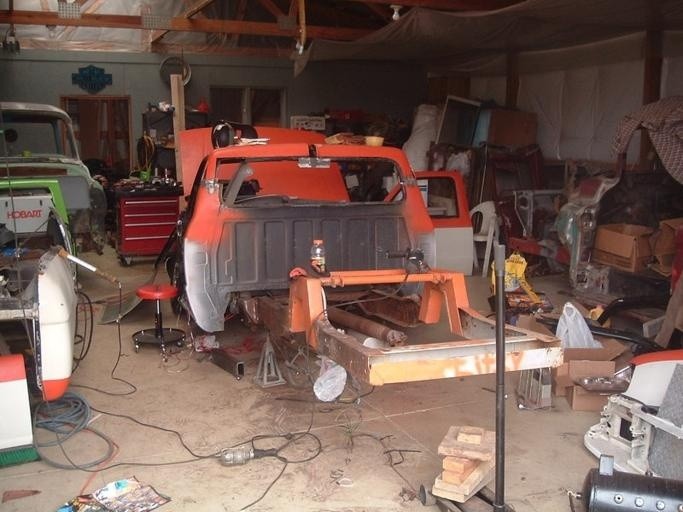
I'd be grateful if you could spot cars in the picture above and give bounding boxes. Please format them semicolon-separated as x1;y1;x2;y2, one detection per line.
0;99;106;245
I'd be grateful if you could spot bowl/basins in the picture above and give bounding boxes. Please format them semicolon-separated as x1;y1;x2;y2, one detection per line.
576;375;629;391
362;136;384;147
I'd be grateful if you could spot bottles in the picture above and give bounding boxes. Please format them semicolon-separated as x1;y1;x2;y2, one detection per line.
151;177;170;184
311;239;326;271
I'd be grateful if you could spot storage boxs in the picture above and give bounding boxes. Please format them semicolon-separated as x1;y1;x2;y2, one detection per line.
593;224;655;272
552;339;625;397
555;360;611;412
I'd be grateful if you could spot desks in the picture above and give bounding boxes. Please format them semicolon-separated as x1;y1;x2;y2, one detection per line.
115;184;183;265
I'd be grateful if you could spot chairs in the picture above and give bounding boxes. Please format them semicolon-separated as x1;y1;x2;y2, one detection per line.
470;201;499;278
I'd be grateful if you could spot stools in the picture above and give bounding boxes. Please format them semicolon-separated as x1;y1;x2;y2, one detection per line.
132;284;186;354
